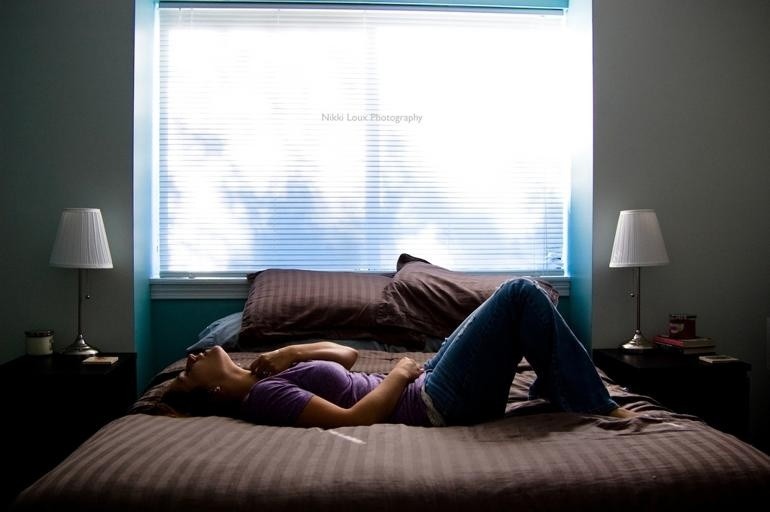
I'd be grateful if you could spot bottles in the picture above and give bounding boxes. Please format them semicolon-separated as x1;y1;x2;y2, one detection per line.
668;313;697;339
23;329;56;357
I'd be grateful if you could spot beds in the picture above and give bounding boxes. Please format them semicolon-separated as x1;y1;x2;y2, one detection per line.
0;346;770;512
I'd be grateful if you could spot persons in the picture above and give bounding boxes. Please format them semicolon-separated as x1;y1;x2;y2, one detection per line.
156;277;648;429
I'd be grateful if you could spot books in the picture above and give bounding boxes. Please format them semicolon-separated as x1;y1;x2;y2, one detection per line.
653;336;718;356
698;353;740;364
81;356;119;365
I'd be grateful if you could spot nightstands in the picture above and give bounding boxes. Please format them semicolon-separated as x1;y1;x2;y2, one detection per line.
594;347;751;448
0;352;138;503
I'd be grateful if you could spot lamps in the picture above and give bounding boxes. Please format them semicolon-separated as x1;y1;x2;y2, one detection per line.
48;207;113;358
609;209;669;353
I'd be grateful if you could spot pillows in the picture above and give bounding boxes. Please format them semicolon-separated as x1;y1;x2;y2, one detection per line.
241;267;427;350
184;310;388;353
376;253;559;340
